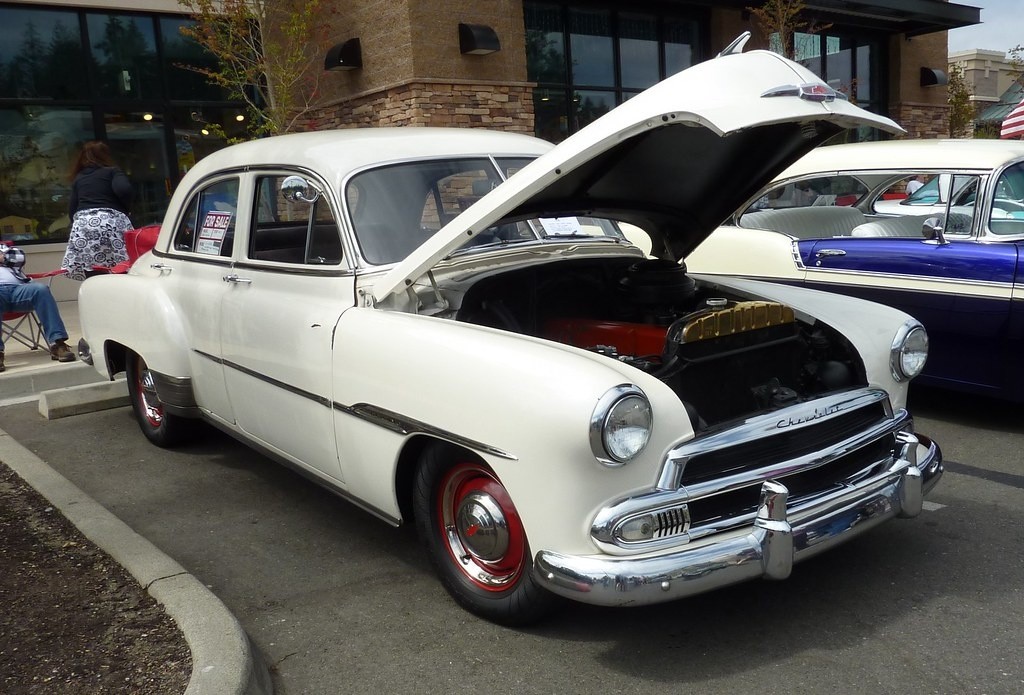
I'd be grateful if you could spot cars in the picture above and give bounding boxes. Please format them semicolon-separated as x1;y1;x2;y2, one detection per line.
558;138;1024;416
74;29;945;631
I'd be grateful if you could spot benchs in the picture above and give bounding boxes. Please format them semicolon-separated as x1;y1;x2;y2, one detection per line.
851;213;973;237
739;206;868;238
218;224;397;265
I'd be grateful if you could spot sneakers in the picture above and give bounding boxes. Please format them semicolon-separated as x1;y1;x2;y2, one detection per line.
51;343;76;362
0;352;5;372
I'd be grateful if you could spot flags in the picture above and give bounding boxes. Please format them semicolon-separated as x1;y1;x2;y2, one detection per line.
1001;99;1024;139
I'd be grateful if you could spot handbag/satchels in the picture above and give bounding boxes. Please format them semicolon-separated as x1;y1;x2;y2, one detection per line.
125;225;161;263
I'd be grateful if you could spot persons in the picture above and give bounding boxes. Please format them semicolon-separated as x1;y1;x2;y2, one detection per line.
906;175;925;196
0;240;76;373
59;142;136;281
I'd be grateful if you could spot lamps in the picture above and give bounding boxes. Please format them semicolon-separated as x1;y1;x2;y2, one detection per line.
324;37;362;71
458;22;501;56
541;89;550;100
921;67;948;86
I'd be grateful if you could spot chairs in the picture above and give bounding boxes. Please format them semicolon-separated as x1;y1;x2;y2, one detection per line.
2;269;69;356
93;225;162;274
